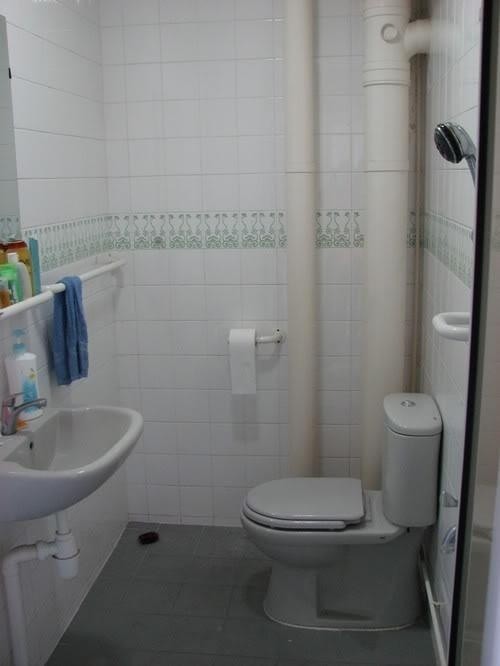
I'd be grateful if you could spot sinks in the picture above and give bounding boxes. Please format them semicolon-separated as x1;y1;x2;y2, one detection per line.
1;405;146;524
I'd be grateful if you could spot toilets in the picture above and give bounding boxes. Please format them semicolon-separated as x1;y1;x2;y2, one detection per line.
241;393;444;631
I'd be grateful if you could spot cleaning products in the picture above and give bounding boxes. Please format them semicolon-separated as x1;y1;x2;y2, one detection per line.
1;240;34;311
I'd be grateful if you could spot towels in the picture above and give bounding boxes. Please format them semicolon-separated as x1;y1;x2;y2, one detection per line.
48;275;88;387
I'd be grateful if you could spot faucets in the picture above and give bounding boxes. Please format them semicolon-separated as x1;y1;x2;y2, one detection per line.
1;393;49;435
